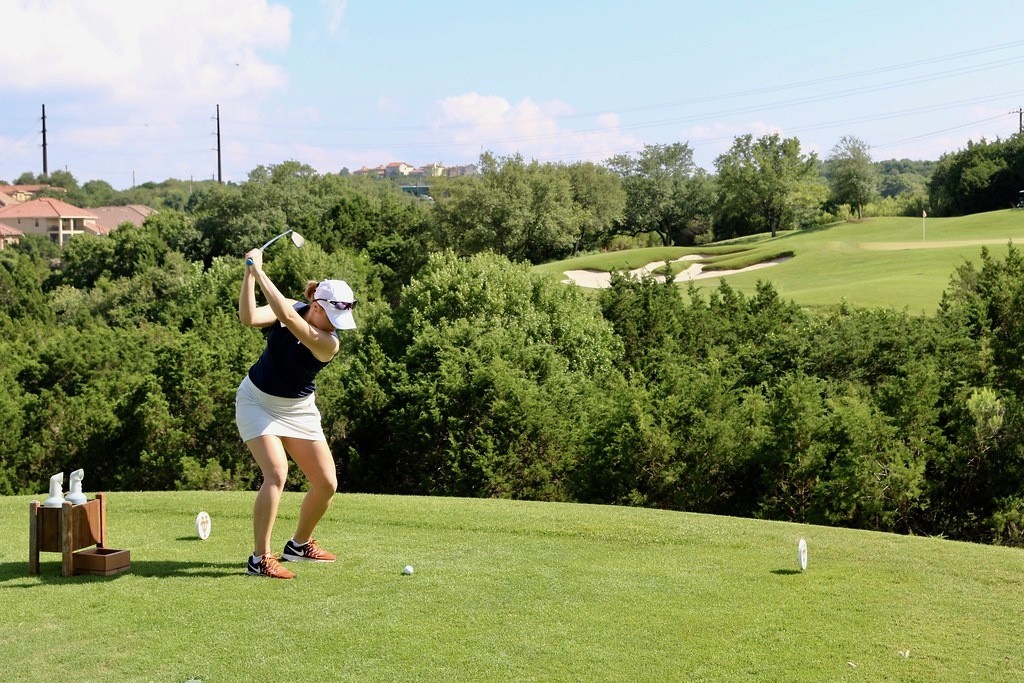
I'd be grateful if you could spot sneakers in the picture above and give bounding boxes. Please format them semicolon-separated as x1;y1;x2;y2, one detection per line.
282;539;337;563
248;552;297;580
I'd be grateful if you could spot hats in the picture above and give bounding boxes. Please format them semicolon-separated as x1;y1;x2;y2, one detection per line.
314;279;357;330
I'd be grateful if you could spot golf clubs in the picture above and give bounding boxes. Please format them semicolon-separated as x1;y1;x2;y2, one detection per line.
245;229;305;266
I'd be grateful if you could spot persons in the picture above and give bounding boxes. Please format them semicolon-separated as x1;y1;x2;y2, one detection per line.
234;247;357;579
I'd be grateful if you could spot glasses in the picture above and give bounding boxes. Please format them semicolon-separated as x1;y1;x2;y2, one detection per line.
312;298;357;310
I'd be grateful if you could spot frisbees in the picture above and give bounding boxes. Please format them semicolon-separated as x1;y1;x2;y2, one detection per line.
195;511;212;540
798;539;808;571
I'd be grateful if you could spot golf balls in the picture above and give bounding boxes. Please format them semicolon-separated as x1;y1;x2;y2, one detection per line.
404;565;414;575
